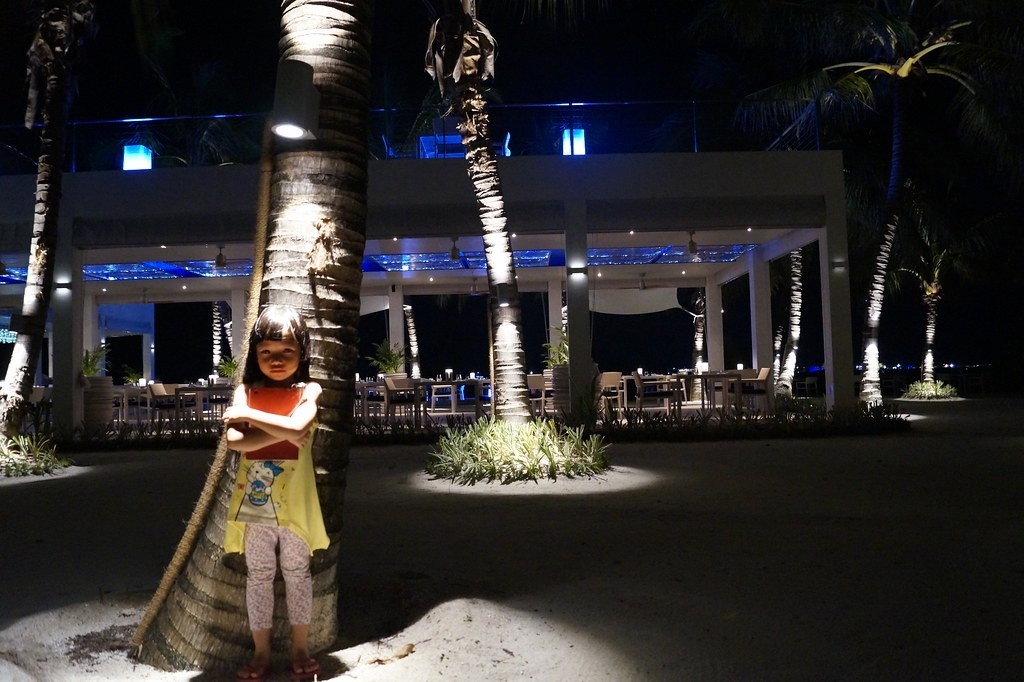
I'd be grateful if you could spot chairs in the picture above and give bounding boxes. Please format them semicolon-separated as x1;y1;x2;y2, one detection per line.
796;377;817;397
42;362;772;434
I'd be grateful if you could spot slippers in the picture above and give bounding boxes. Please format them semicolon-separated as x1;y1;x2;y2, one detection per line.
235;662;271;682
290;657;321;680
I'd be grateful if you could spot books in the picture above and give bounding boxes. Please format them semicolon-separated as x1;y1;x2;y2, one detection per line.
244;387;299;460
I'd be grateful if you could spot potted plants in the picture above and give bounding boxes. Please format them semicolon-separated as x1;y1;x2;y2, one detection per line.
367;340;410;396
544;325;570;410
83;343;116;426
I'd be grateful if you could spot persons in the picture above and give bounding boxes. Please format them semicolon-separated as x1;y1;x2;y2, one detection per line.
221;305;331;682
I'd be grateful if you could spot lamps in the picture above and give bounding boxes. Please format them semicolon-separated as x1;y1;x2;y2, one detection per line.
688;232;697;254
470;277;481;295
639;273;647;292
216;243;226;267
450;236;460;260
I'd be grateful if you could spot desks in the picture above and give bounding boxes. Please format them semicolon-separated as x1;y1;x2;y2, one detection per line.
115;386;141;429
664;374;741;422
414;380;483;429
174;386;235;429
623;375;665;408
354;379;385;424
